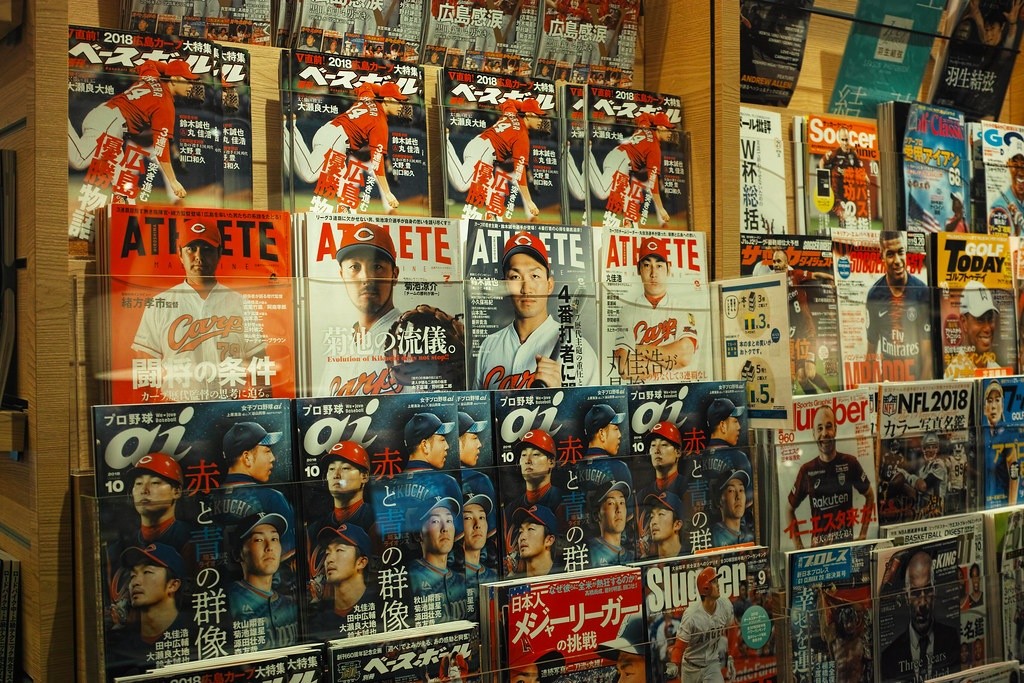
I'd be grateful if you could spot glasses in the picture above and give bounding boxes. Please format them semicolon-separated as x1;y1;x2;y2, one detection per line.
840;138;848;142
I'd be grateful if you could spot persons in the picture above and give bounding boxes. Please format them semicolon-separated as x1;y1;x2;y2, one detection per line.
63;21;1023;683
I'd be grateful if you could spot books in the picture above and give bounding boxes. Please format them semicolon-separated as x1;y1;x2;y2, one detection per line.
741;0;1024;683
63;0;779;683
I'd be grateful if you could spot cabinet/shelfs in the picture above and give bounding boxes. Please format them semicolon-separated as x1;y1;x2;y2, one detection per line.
0;0;1024;683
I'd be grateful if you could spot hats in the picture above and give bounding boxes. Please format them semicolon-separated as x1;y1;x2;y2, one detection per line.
404;413;455;448
960;280;1000;317
1003;139;1024;165
164;60;201;80
379;83;407;101
415;495;460;521
521;99;546;116
120;543;186;579
458;411;488;437
317;523;372;560
232;512;288;552
502;231;550;271
177;218;221;248
223;422;283;459
491;382;1003;683
639;237;667;264
126;453;183;487
463;492;493;514
335;222;397;264
652;113;675;128
320;440;370;473
